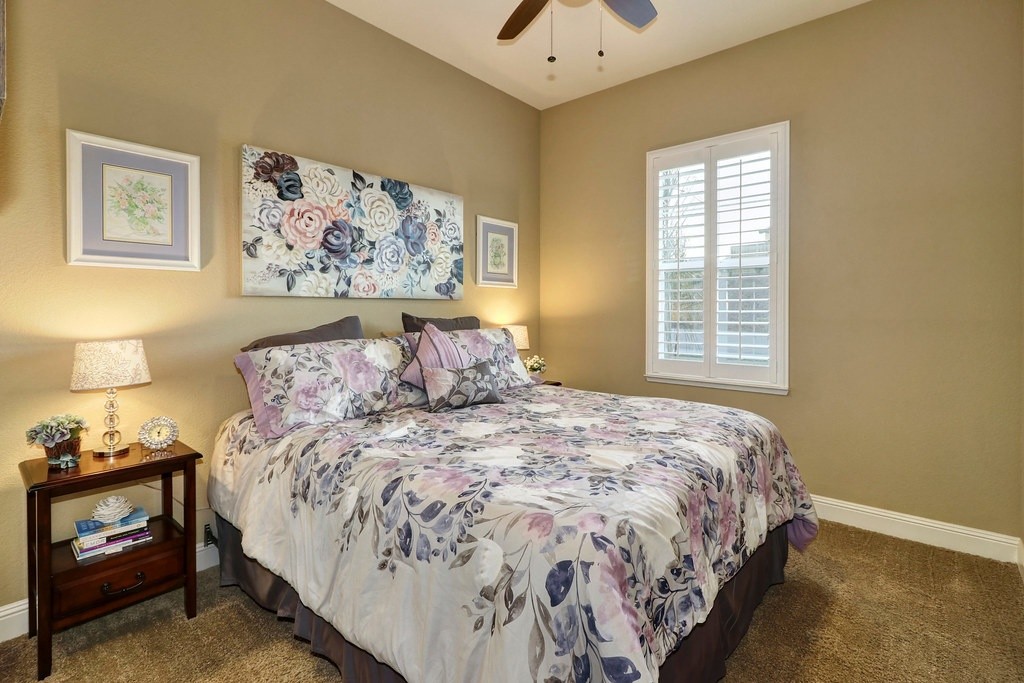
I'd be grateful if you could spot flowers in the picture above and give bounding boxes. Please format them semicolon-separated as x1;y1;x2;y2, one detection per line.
26;414;88;448
522;354;546;374
49;452;81;468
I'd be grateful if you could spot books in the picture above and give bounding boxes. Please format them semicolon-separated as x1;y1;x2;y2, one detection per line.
69;506;154;562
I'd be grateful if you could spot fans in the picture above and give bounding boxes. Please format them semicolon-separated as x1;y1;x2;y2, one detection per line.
497;0;658;39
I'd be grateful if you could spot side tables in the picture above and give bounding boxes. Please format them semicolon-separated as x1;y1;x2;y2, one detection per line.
543;380;562;386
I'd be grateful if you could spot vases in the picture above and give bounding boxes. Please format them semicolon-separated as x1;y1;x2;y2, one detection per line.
41;436;82;459
530;372;544;384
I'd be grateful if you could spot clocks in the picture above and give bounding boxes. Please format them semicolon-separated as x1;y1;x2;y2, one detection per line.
138;415;179;453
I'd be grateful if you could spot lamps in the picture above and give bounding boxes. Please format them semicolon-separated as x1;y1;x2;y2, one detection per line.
70;339;152;458
500;325;530;349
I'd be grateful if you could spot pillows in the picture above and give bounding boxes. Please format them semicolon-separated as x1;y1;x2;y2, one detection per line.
420;360;505;414
232;335;429;439
405;327;546;391
401;311;481;332
399;322;475;392
240;314;365;352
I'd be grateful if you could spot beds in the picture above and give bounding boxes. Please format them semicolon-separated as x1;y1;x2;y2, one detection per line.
204;386;820;683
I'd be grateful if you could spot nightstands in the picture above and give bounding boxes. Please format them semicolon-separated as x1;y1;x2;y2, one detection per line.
17;440;203;682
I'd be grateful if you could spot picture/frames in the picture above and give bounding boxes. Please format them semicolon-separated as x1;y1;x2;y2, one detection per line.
476;215;518;290
65;129;202;272
